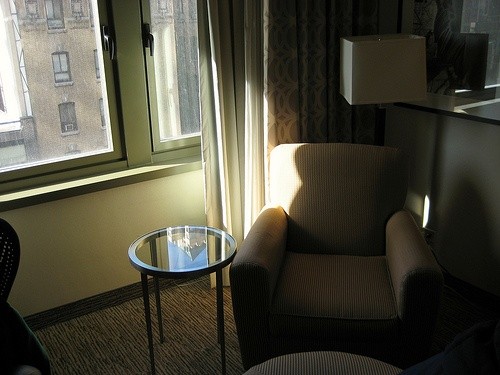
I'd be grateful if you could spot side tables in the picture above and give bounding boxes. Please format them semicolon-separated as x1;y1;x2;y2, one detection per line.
128;225;237;375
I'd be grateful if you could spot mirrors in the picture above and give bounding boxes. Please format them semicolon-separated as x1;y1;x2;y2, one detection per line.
398;0;500;101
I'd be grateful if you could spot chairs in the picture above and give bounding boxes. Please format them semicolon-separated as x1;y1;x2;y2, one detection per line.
0;219;52;375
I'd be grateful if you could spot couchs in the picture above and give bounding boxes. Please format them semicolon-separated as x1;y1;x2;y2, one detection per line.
229;142;442;372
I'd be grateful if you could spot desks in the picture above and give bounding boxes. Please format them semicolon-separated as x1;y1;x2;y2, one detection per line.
382;93;500;316
241;351;404;375
